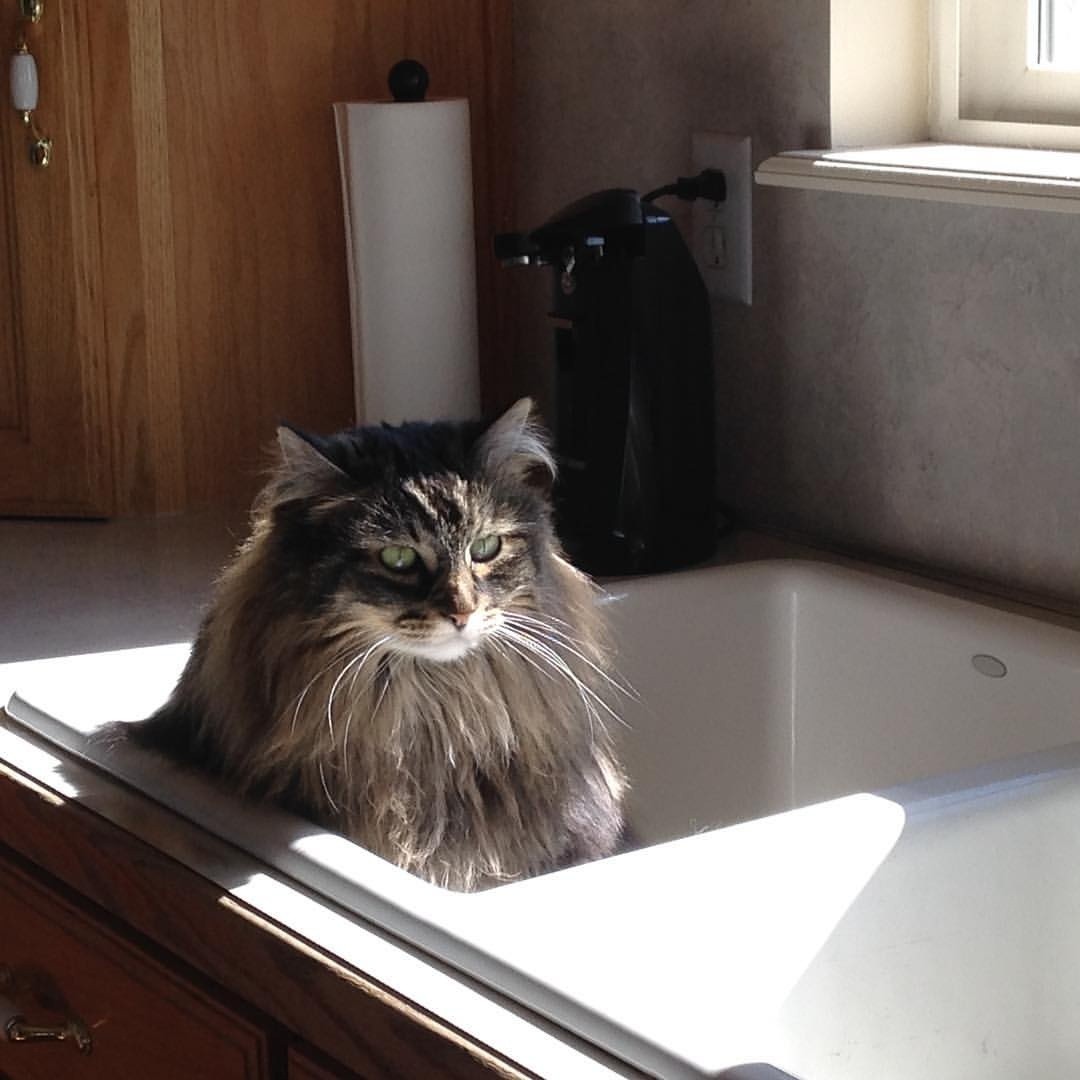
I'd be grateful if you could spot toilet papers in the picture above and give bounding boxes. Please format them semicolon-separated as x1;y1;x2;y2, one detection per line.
333;96;482;428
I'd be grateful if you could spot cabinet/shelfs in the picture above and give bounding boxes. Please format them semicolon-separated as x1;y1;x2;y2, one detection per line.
0;778;510;1080
0;0;517;534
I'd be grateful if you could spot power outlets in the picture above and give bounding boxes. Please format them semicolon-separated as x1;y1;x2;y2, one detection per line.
681;132;756;313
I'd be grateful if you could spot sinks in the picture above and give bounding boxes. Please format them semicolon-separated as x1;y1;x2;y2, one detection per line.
3;556;1080;904
447;751;1080;1080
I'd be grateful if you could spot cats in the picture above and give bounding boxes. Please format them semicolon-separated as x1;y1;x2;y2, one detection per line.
86;397;649;893
